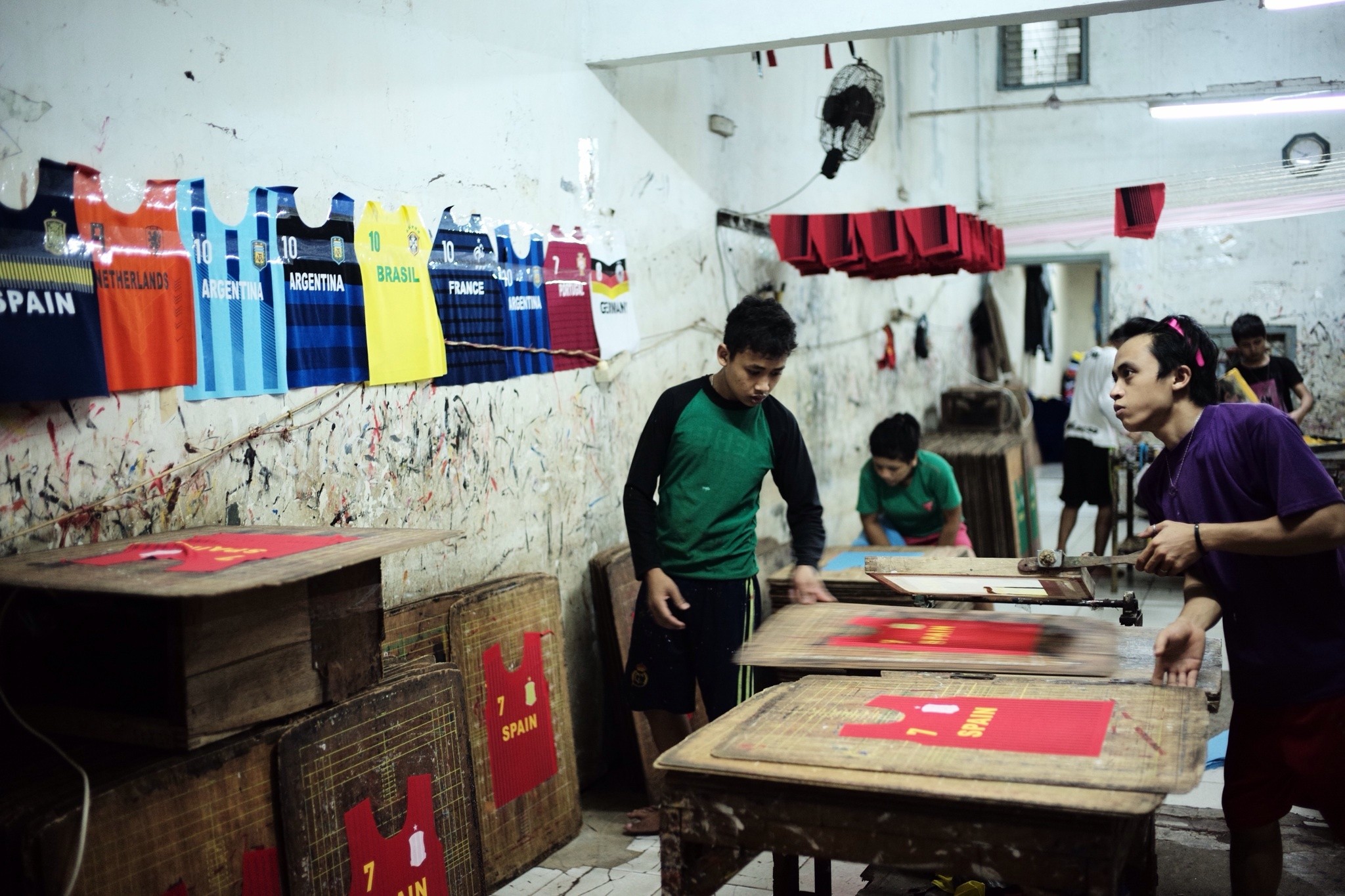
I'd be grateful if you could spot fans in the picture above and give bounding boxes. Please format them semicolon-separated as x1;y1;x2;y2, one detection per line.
819;39;885;180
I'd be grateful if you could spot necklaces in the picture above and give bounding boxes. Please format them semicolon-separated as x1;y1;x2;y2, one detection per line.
1162;406;1207;500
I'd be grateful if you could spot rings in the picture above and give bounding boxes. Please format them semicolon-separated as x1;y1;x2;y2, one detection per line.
1152;524;1157;535
1158;567;1169;573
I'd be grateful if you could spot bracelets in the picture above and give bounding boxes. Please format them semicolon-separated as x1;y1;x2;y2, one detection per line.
1194;522;1210;558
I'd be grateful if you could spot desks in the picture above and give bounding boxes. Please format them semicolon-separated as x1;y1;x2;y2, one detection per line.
653;680;1170;896
733;605;1121;682
1107;455;1169;593
0;530;467;754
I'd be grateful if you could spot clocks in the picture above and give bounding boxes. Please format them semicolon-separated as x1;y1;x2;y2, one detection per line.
1281;131;1331;178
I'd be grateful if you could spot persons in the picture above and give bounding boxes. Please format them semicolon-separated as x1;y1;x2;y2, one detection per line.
1225;314;1317;426
1108;313;1345;896
857;411;977;560
1053;326;1144;556
613;295;840;834
